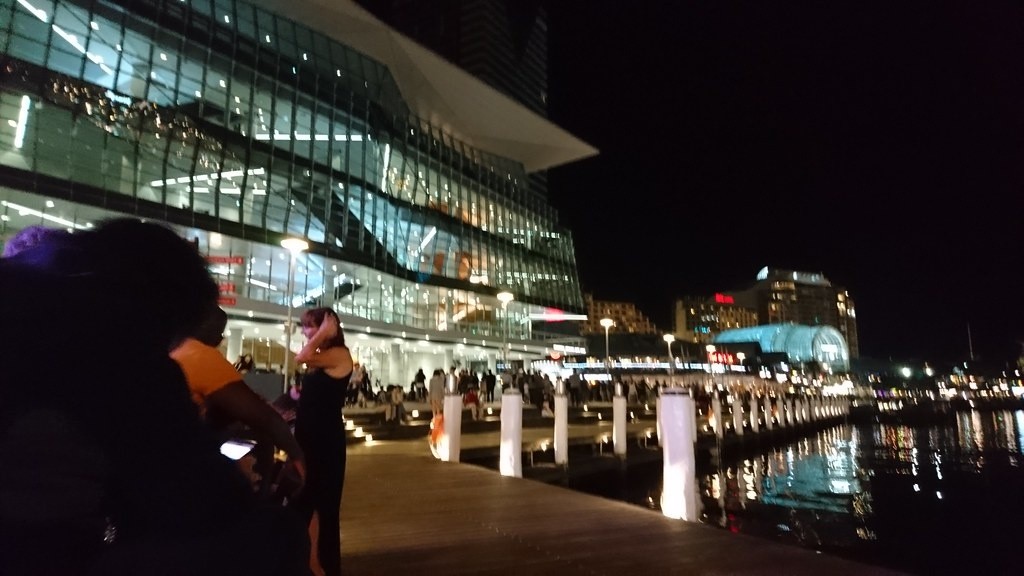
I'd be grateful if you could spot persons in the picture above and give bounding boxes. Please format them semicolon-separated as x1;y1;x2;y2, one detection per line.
167;303;305;500
0;218;270;576
288;307;353;576
234;352;720;433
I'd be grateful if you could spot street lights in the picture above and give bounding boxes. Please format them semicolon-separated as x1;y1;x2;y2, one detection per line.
497;292;514;372
599;317;614;374
279;236;311;396
705;344;716;377
661;333;676;373
737;352;746;376
